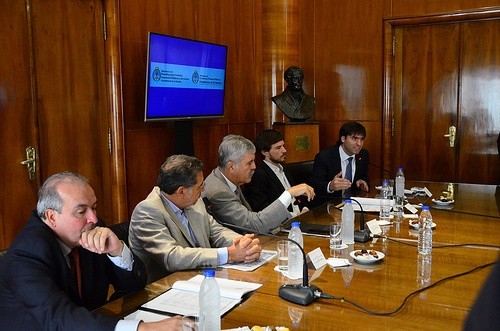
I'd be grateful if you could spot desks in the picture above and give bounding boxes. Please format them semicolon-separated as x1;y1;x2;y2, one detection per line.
89;179;500;331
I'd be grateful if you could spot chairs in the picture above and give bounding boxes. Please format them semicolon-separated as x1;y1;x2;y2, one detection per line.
287;159;336;206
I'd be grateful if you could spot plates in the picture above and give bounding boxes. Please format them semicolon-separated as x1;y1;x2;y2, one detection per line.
432;206;453;209
431;199;455;206
409;231;436;235
410;220;437;230
350;250;386;263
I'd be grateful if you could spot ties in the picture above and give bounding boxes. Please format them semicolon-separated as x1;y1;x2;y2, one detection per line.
344;157;353;199
235;189;240;200
68;252;80;305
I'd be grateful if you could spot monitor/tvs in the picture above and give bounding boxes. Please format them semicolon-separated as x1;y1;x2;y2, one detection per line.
144;30;228;122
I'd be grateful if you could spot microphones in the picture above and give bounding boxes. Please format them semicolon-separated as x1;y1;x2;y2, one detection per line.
316;195;371;242
367;160;396;199
201;197;322;305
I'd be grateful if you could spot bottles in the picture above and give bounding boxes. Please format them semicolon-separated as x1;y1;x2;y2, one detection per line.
199;270;222;331
380;181;390;217
288;301;302;328
342;199;355;244
342;244;354;286
418;206;434;254
288;221;303;279
416;256;431;300
396;168;405;196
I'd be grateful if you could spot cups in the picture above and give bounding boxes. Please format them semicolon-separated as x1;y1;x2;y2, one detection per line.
181;314;205;331
393;195;403;212
276;239;288;270
330;222;343;248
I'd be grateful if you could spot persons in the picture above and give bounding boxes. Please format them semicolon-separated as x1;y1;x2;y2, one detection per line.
271;66;316;121
204;135;316;237
246;131;313;224
128;154;262;285
0;172;199;331
307;121;370;206
